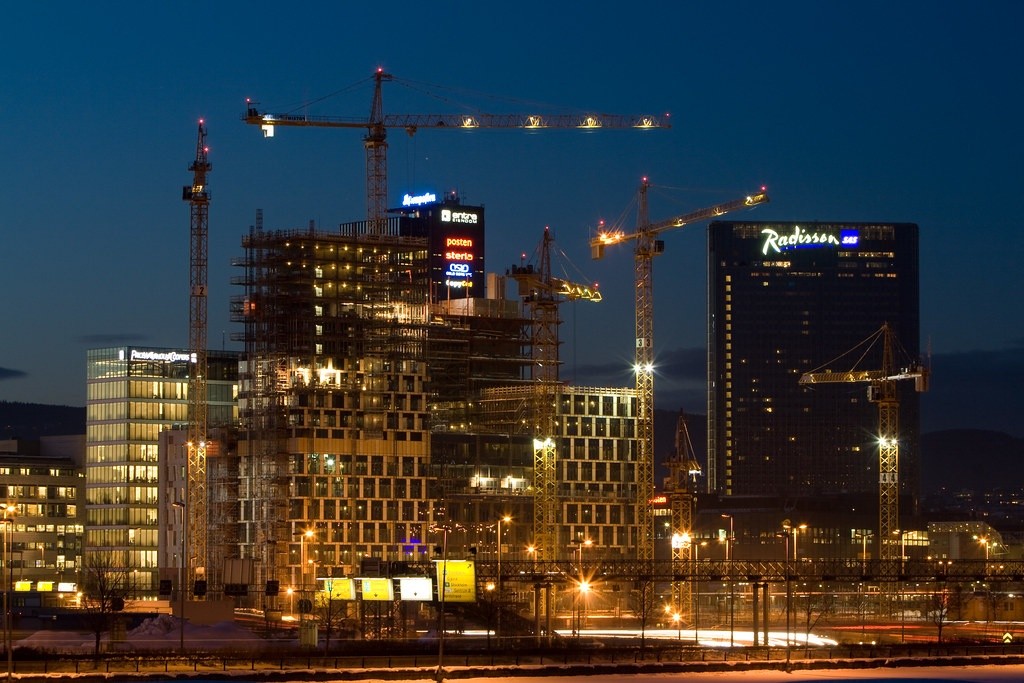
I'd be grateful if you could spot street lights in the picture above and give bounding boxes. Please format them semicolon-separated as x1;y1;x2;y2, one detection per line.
973;535;998;560
855;532;874;574
432;526;448;682
939;562;952;575
301;531;315;590
578;539;592;565
38;545;45;568
134;570;138;599
171;500;186;652
0;519;14;681
577;584;592;642
4;506;16;652
288;589;293;620
782;523;808;559
497;516;513;647
777;533;790;562
893;530;915;574
721;514;735;651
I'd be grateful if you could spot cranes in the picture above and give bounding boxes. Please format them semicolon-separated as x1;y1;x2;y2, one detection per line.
587;173;771;615
240;64;674;234
181;116;215;593
660;409;704;628
504;226;604;616
797;319;930;621
